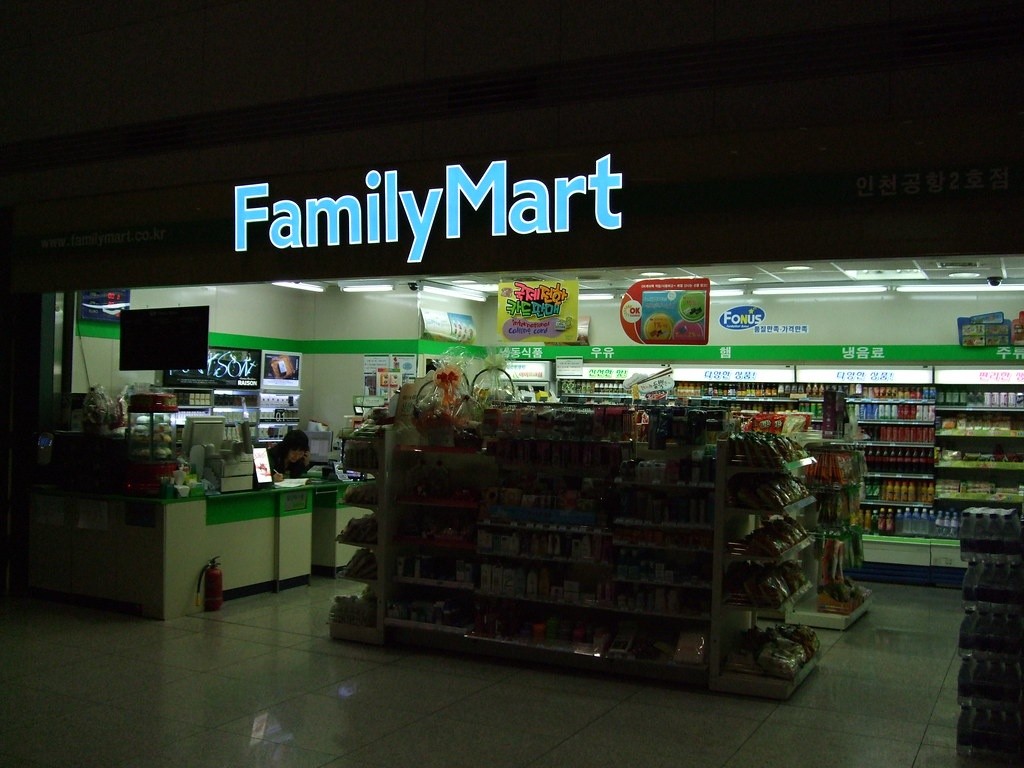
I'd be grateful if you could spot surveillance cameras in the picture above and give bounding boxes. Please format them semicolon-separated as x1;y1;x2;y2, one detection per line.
409;283;418;290
987;277;1002;286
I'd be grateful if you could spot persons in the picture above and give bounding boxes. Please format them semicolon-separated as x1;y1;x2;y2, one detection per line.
253;429;311;491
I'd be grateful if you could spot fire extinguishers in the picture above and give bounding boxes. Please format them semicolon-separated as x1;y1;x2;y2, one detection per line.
195;555;224;612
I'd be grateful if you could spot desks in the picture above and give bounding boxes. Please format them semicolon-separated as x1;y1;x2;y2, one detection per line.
28;471;373;621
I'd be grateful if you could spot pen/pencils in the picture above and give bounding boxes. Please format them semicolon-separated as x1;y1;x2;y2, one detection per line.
271;466;279;474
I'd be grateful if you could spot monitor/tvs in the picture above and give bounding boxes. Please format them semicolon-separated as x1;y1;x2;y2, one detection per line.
120;306;209;370
182;418;225;460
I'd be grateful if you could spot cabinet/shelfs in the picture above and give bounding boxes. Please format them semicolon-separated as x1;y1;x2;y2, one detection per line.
329;391;1024;702
